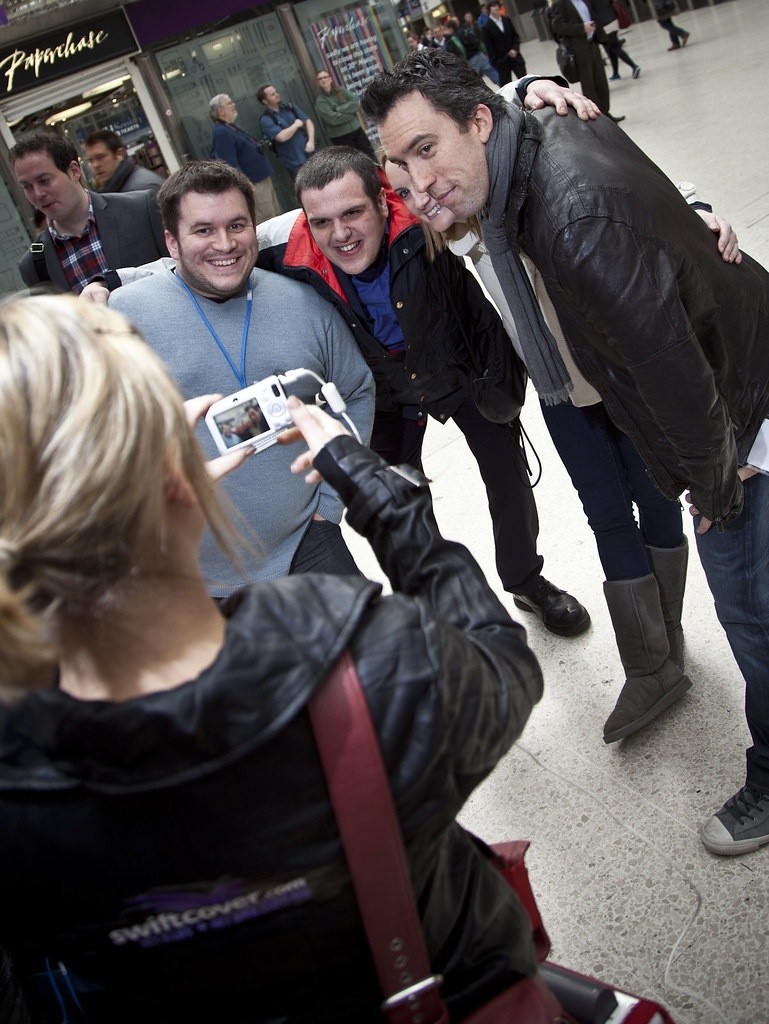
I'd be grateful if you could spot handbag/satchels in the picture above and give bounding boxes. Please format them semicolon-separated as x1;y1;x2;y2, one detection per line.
556;38;581;84
311;649;674;1024
612;0;631;29
445;278;541;487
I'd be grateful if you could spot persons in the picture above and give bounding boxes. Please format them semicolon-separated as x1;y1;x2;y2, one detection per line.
84;129;165;193
0;293;544;1024
381;154;743;742
106;159;377;607
222;405;270;449
208;93;283;226
7;133;171;298
405;0;527;87
359;48;769;854
90;74;602;633
315;70;378;164
650;0;690;50
256;85;316;180
549;0;640;121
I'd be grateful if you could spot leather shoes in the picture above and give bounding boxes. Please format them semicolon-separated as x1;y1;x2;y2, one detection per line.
514;573;590;638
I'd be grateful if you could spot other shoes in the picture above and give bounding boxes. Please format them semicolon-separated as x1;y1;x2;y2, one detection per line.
683;32;689;48
668;43;680;51
610;74;619;79
633;66;641;79
608;114;625;123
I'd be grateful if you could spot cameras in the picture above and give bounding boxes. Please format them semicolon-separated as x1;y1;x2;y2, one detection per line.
202;375;296;458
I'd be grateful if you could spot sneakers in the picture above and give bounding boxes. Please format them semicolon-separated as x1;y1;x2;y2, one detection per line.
699;785;769;856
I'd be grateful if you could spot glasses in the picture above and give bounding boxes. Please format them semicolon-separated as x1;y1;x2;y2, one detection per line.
316;75;330;81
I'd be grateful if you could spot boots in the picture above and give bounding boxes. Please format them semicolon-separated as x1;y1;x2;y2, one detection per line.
602;570;693;744
644;534;689;672
